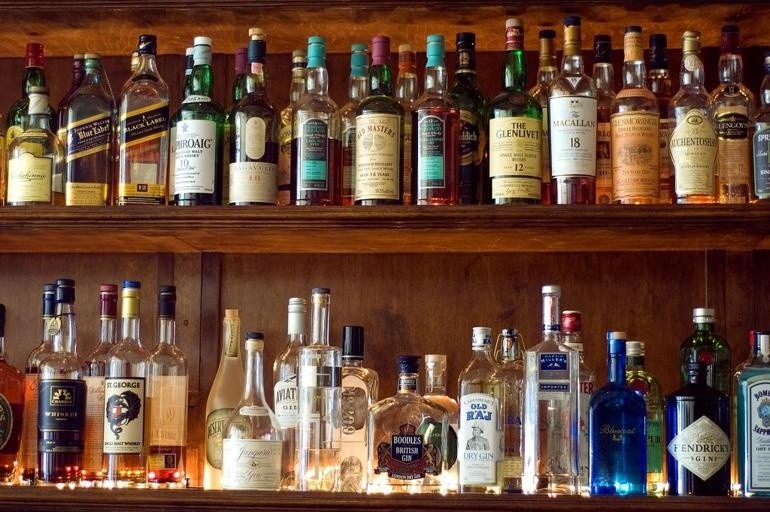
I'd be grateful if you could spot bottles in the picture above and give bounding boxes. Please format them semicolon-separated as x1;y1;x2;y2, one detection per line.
368;354;450;493
677;306;734;497
395;43;420;206
730;330;756;497
590;33;617;204
7;41;58;208
667;29;719;204
587;330;648;498
546;310;599;498
421;355;460;493
709;54;757;204
448;32;491;205
341;325;380;492
204;308;245;489
663;361;733;499
340;43;371;205
120;48;147;206
59;52;86;209
524;284;581;497
85;284;120;487
175;36;225;207
546;15;599;204
0;110;7;208
143;283;190;489
65;53;115;208
625;340;669;498
103;279;151;489
292;35;342;205
170;47;195;207
747;56;770;204
494;327;527;494
22;283;57;486
120;33;169;207
526;28;561;204
355;35;406;205
0;302;24;483
279;48;307;206
411;34;461;205
274;298;309;492
225;46;248;207
647;33;677;203
737;332;770;497
485;17;544;204
38;278;88;488
7;87;61;209
457;326;507;494
222;332;282;492
297;286;343;492
235;28;279;207
610;26;659;204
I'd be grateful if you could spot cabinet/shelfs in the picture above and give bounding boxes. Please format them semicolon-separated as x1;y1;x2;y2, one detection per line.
1;0;770;512
